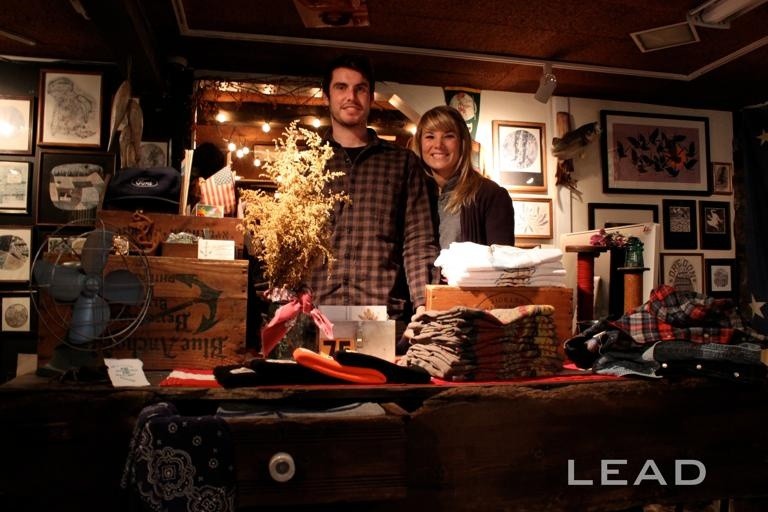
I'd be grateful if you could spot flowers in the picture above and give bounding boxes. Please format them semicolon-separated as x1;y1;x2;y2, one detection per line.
235;118;357;291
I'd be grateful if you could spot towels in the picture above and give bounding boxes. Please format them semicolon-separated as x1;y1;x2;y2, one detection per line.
403;241;567;382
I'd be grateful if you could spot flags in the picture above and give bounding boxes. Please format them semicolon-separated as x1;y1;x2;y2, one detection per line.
202;164;237;218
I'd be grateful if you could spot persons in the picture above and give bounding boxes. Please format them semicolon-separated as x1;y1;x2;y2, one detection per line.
47;79;95;142
301;53;442;336
405;107;515;286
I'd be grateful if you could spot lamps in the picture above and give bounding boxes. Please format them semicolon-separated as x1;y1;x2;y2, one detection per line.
534;64;557;104
689;0;768;30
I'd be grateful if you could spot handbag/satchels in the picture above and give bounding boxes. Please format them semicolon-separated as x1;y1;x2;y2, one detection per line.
104;166;181;214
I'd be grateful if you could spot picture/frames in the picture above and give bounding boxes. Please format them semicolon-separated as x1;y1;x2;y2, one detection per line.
586;109;738;298
490;118;554;241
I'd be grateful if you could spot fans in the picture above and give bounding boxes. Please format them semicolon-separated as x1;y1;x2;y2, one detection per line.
28;218;155;386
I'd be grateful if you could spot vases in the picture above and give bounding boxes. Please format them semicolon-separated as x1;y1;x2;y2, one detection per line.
262;277;314;363
